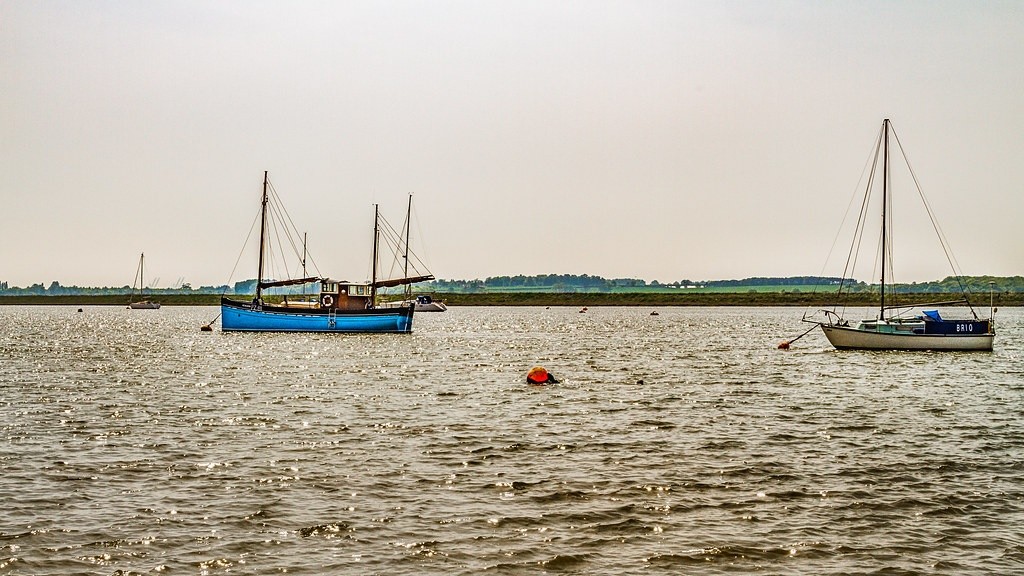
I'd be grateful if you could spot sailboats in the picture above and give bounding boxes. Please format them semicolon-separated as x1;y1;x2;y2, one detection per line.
219;170;437;334
126;251;161;309
379;191;449;312
800;118;997;351
